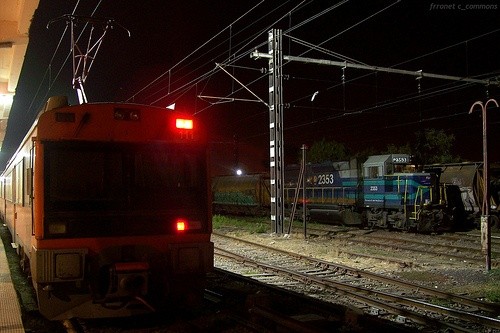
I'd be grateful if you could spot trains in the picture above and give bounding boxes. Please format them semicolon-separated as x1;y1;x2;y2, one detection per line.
0;93;214;324
215;151;479;234
